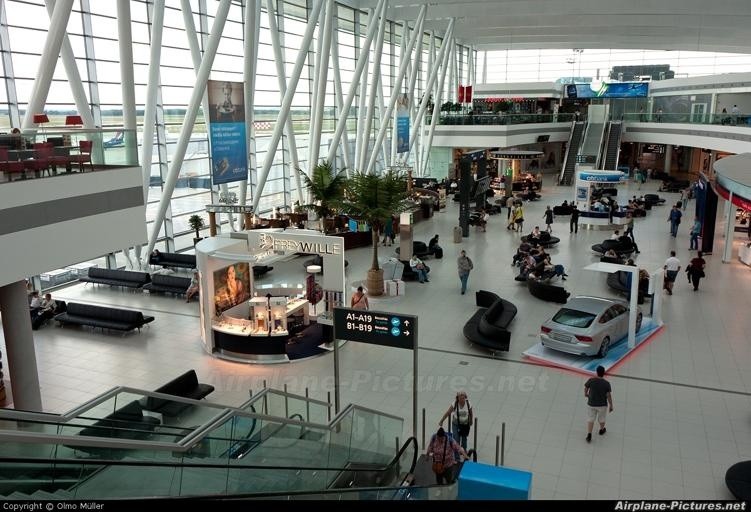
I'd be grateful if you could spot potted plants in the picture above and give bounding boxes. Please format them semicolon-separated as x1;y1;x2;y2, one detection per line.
292;158;417;297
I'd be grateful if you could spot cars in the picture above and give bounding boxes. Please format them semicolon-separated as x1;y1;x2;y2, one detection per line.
539;294;644;360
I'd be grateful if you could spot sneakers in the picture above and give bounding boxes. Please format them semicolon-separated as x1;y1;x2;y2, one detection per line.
586;438;591;441
511;262;520;266
599;428;605;435
420;279;430;283
507;227;522;232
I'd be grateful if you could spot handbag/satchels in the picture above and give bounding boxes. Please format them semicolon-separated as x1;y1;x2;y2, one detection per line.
416;264;424;269
432;464;444;473
515;218;524;223
458;424;469;436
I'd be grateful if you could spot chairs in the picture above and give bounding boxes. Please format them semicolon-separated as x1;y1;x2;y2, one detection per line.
0;134;94;181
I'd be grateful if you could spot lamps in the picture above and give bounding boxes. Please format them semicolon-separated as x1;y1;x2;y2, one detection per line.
32;113;50;141
65;116;83;145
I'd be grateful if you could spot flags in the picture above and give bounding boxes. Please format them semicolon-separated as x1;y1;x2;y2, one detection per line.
459;86;465;104
466;86;472;103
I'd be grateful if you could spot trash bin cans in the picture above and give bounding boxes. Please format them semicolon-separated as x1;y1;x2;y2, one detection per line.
455;226;463;243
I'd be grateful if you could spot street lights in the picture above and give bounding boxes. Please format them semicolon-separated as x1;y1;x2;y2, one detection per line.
306;262;321;317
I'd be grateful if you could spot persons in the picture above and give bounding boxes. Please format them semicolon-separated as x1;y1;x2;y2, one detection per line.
638;106;646;122
186;270;200;304
410;253;431;284
656;105;665;124
31;294;58;330
439;390;475;464
28;290;44;314
721;107;729;125
428;234;445;260
150;264;176;277
536;106;543;122
457;249;475;296
350;286;370;310
226;264;245;306
730;104;744;126
425;427;470;485
498;164;708;296
583;365;615;443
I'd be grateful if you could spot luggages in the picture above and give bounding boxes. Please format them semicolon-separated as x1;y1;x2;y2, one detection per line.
435;247;443;258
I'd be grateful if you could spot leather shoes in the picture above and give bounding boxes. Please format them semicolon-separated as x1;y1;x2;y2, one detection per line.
562;274;568;280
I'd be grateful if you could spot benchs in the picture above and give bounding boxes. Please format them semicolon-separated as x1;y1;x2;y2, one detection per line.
591;239;651;304
79;252;199;300
521;234;570;304
63;367;216;460
394;239;434;282
29;299;155;338
463;289;517;357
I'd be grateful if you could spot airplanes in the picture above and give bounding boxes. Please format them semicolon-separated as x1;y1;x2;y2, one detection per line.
69;128;125;150
